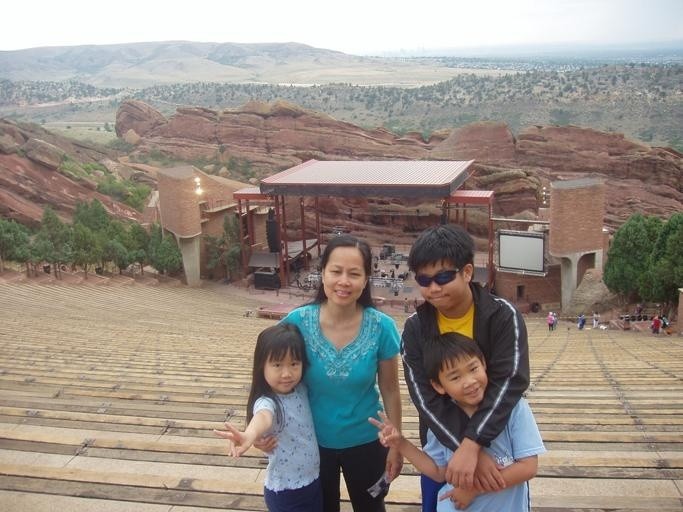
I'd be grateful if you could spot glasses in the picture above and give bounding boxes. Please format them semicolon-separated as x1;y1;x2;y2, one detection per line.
413;263;466;288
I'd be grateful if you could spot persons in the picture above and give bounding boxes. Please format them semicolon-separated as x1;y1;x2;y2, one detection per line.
398;222;532;512
412;297;418;310
365;330;550;512
545;300;675;337
210;321;325;512
278;233;404;512
404;297;409;313
